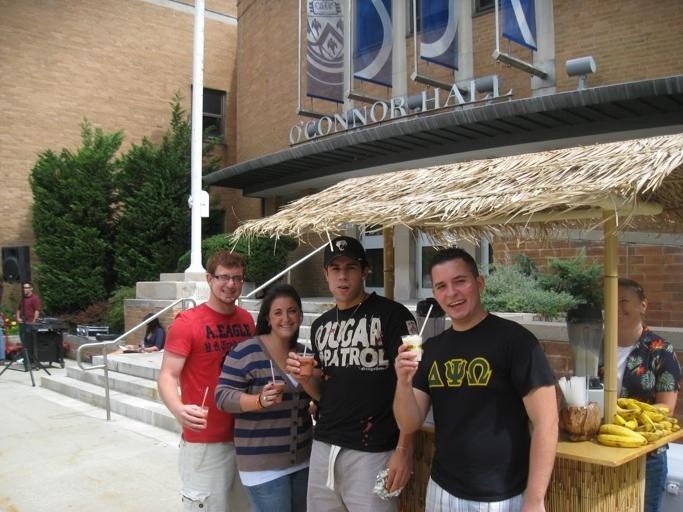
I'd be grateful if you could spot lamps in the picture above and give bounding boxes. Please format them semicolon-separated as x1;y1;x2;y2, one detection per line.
307;122;320;137
340;109;367;129
565;57;596;90
392;93;427;118
454;75;503;105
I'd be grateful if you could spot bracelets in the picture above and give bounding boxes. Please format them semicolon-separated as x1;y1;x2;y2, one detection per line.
256;394;265;409
396;444;414;462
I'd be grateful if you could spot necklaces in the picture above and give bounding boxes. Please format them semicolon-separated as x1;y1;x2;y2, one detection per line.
336;294;367;343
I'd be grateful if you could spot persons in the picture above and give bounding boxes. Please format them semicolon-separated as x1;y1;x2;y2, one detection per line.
285;236;419;512
598;278;681;512
392;248;560;512
214;284;312;512
158;251;256;512
109;312;164;354
16;282;41;363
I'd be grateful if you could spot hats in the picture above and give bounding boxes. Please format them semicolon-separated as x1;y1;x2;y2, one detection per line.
323;236;366;268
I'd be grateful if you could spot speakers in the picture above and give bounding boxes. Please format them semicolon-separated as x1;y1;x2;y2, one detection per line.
2;245;29;284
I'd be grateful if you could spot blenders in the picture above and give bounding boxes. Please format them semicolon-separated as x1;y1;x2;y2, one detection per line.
415;298;445;343
566;300;605;420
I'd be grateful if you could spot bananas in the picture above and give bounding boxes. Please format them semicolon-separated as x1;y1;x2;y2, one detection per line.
597;398;681;448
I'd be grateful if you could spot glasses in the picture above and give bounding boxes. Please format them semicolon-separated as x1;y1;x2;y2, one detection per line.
23;287;30;289
215;275;245;283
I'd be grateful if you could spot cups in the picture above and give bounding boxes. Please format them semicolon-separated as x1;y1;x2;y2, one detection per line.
297;353;315;376
267;380;285;404
400;334;422;362
194;406;209;429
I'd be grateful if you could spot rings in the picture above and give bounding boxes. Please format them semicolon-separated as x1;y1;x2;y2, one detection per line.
263;396;267;401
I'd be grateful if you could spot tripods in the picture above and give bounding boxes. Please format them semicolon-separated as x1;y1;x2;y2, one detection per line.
0;283;52;387
